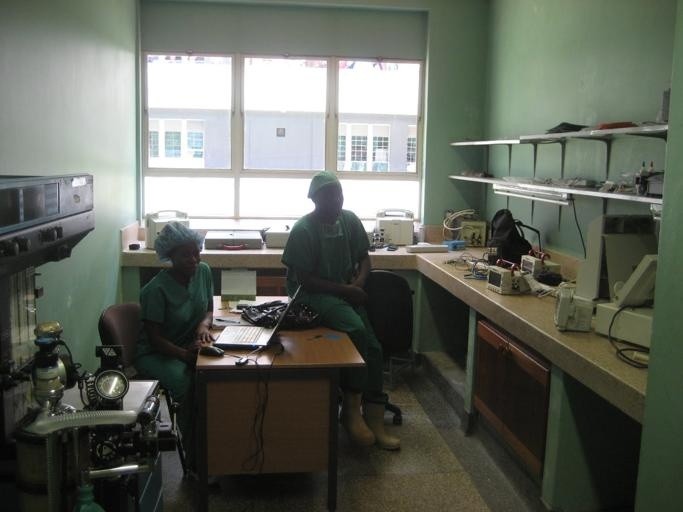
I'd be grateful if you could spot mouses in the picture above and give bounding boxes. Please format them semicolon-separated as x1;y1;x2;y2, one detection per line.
199;346;224;357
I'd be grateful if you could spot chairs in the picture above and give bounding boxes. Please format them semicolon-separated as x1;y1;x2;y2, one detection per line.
353;269;413;426
99;302;187;476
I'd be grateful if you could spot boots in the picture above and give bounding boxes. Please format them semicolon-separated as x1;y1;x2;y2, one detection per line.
340;392;376;446
362;401;401;450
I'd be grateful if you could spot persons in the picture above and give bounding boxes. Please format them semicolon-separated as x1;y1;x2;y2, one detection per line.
281;171;401;451
133;220;215;485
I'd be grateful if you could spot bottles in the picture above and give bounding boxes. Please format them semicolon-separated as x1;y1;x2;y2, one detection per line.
648;160;655;176
374;233;380;245
639;161;648;190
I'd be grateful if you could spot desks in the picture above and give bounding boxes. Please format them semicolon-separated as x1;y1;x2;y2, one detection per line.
194;294;365;512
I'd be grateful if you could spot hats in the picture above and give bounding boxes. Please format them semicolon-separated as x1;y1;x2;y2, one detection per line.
307;170;338;198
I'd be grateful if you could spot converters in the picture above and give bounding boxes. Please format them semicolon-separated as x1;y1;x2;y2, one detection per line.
235;357;249;365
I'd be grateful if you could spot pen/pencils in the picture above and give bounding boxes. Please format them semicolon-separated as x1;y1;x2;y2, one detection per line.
216;319;240;324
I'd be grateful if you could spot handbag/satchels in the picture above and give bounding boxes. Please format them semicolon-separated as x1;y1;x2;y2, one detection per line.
241;300;319;331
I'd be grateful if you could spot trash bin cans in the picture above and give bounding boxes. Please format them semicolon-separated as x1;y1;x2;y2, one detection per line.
12;426;66;496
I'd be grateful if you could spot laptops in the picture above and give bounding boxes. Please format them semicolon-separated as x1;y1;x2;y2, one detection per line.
212;286;303;350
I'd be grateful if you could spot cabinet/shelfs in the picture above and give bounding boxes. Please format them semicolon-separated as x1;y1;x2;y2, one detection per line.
474;319;549;485
447;122;667;233
